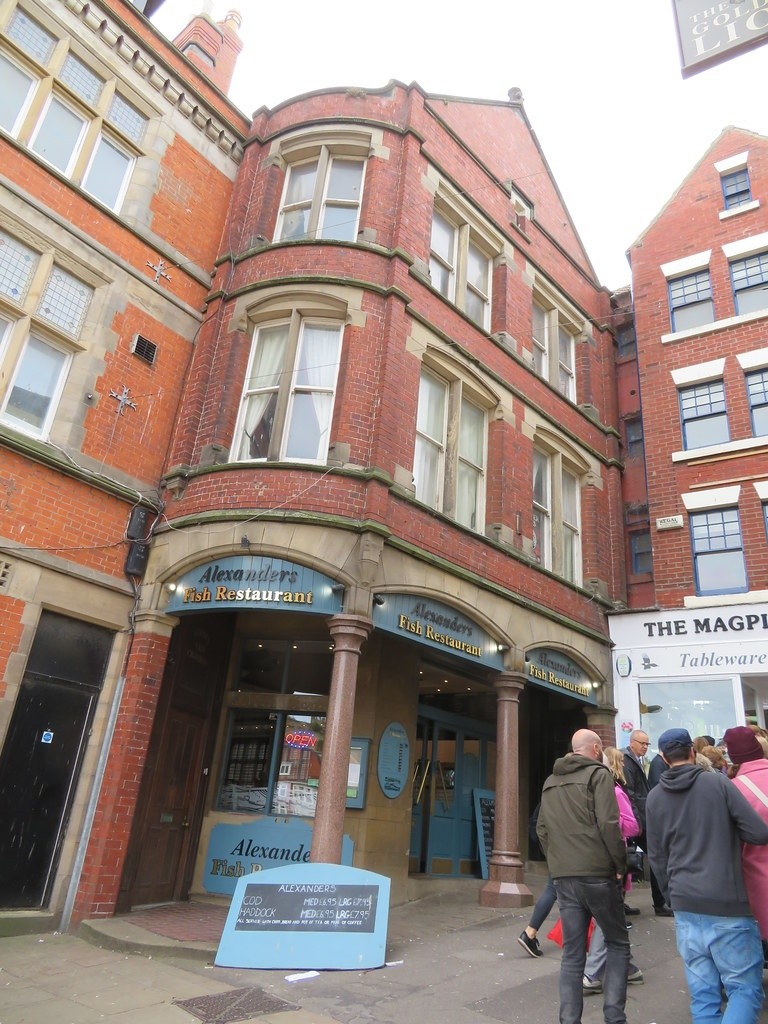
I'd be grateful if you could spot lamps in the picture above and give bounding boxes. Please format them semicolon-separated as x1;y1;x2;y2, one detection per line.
498;645;509;652
331;584;345;594
240;534;251;550
373;594;385;606
164;582;177;593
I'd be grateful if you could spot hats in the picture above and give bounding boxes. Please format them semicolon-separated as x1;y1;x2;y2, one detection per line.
658;728;695;753
723;726;764;764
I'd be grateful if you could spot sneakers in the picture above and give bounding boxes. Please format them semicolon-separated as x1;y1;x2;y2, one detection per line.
518;930;543;957
627;969;643;984
583;973;603;995
627;921;632;928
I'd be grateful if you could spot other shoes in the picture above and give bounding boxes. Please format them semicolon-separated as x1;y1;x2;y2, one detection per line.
624;902;640;915
652;903;674;917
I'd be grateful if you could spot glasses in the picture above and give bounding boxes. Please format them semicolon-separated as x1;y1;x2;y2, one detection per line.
632;739;651;747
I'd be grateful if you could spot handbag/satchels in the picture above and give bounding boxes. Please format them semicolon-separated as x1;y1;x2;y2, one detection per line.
625;847;643;873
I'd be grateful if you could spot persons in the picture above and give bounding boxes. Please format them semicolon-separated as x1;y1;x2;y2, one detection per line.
517;726;768;1024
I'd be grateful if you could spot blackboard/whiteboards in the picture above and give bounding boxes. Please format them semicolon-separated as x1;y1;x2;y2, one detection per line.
474;787;497;880
